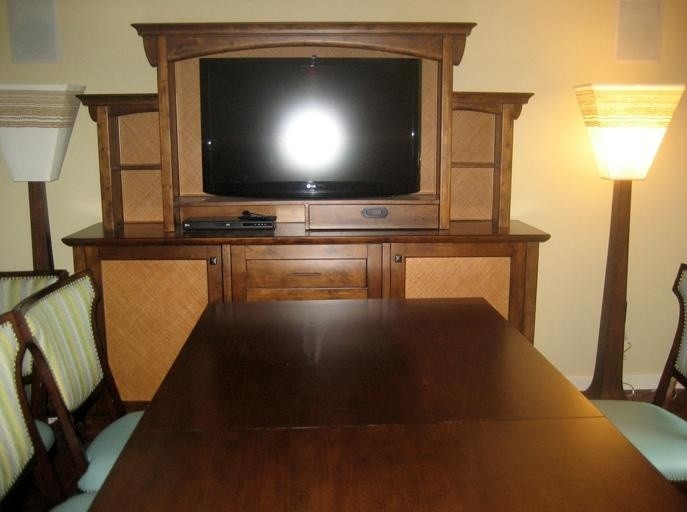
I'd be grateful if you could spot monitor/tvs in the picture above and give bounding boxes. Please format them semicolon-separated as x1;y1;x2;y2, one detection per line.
199;56;421;201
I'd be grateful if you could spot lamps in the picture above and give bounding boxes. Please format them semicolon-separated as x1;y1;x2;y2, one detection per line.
574;82;687;397
0;83;89;270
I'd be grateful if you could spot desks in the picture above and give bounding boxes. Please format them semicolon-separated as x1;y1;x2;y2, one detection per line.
85;296;687;509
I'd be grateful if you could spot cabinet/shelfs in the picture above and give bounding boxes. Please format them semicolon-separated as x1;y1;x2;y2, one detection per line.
59;23;549;416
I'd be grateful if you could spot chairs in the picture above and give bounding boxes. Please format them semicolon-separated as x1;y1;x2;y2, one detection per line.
585;263;687;494
0;270;145;511
